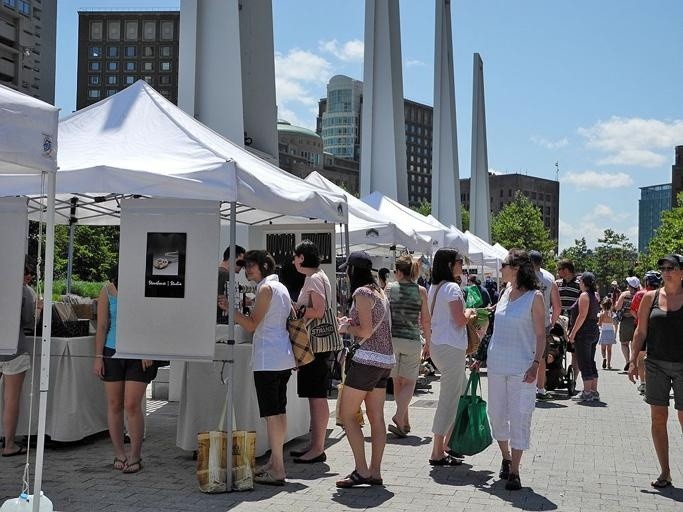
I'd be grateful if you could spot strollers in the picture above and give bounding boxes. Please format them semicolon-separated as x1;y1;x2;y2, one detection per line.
544;314;576;396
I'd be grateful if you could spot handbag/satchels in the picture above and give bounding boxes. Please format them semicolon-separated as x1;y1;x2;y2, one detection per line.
447;366;493;455
612;297;633;323
334;354;365;427
307;273;344;355
429;280;482;356
277;278;316;368
345;288;387;375
195;380;255;493
465;284;483;309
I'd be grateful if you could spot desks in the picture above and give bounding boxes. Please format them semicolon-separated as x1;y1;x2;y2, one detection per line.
0;335;111;450
175;340;312;462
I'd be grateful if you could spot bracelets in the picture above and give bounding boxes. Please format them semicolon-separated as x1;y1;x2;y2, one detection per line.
532;358;539;365
96;355;103;358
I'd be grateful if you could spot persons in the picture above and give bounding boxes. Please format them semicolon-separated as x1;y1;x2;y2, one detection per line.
93;267;158;474
613;276;640;372
0;266;41;456
486;279;511;307
471;253;546;490
294;239;340;463
628;254;683;487
336;250;393;487
569;271;600;401
460;274;489;309
632;271;663;393
600;281;619;305
600;298;618;370
529;249;562;403
218;244;250;323
426;248;477;464
219;248;297;488
553;258;581;393
385;254;431;437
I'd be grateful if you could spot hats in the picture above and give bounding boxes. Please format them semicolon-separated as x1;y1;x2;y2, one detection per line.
581;272;596;286
626;275;640;290
338;251;374;270
656;253;683;268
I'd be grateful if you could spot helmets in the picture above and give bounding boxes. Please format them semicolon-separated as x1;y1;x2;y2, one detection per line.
643;269;662;288
236;249;276;277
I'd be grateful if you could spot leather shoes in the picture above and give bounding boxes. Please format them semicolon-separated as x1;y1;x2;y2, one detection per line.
295;453;329;464
291;450;306;455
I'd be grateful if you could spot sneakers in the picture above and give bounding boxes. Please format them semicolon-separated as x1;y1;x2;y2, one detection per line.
572;391;595;402
602;358;604;368
506;472;521;490
500;459;510;476
535;392;555;401
639;384;646;392
591;390;599;400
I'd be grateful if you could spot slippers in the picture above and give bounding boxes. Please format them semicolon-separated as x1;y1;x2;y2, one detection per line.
430;456;462;467
653;476;673;491
123;460;143;475
336;469;377;488
387;424;408;440
113;456;126;470
372;478;384;487
1;441;30;458
252;467;288;487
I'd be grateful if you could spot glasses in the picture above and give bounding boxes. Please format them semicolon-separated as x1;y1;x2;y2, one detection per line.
501;262;513;268
658;264;675;273
289;254;299;261
393;270;398;273
455;258;465;266
24;266;37;277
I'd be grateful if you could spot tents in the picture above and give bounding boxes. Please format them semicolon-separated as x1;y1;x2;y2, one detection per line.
2;82;59;507
290;171;518;276
13;78;347;491
